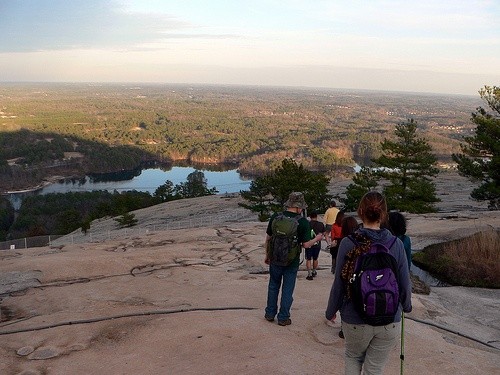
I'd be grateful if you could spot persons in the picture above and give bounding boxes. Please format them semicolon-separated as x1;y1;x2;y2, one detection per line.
324;201;341;249
264;192;324;326
387;212;411;274
303;212;324;281
325;191;413;375
330;212;347;275
341;217;359;236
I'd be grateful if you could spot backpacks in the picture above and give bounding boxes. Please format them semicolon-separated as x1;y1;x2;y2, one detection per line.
310;221;319;246
267;212;303;270
343;231;401;320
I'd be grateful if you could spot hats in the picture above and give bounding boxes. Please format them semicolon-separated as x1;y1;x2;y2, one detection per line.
284;192;309;210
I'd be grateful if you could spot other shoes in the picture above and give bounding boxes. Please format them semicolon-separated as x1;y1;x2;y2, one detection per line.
264;314;274;322
306;275;313;280
312;271;317;276
277;319;291;326
